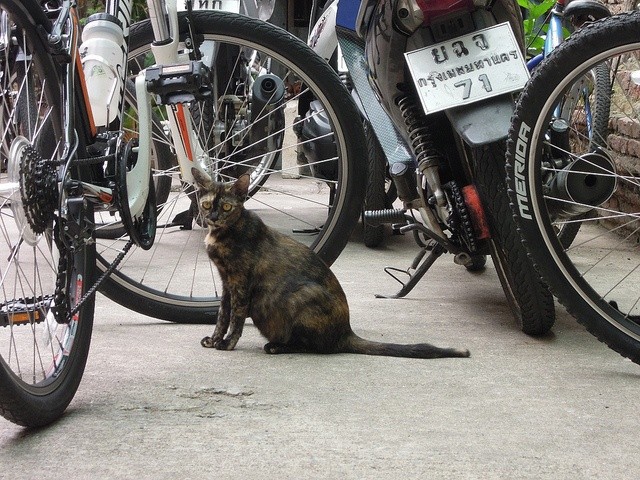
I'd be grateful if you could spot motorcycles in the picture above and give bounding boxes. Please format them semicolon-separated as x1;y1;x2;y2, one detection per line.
284;0;559;338
173;0;281;201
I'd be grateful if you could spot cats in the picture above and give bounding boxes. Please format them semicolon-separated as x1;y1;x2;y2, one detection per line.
190;166;472;359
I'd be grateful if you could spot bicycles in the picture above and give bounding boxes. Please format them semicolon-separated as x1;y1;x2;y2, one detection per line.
0;4;370;429
525;0;616;251
508;11;640;364
